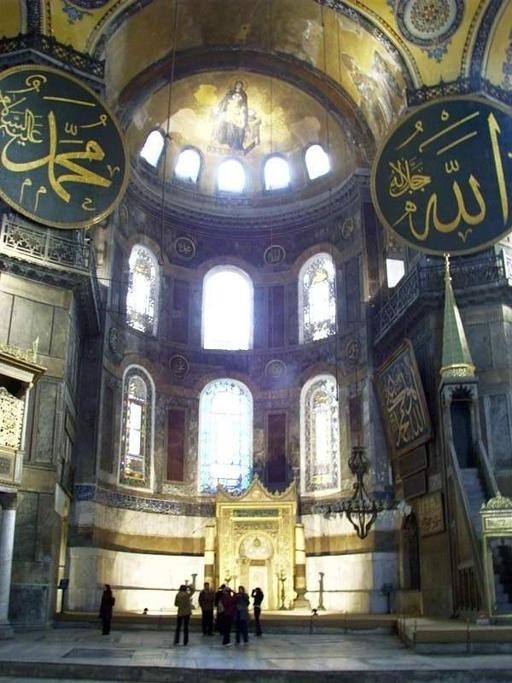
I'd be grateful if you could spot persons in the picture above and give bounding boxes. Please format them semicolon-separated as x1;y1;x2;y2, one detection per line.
199;582;214;636
215;79;247;149
251;588;264;636
215;584;250;646
99;585;112;635
174;585;194;645
